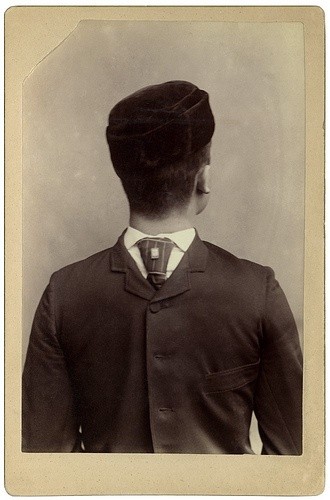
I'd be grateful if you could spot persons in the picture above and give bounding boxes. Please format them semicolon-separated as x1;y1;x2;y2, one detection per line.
21;79;303;455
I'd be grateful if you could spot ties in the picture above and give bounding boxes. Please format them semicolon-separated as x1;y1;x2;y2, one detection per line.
137;238;174;289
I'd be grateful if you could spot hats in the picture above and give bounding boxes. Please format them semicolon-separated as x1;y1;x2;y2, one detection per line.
106;80;215;169
